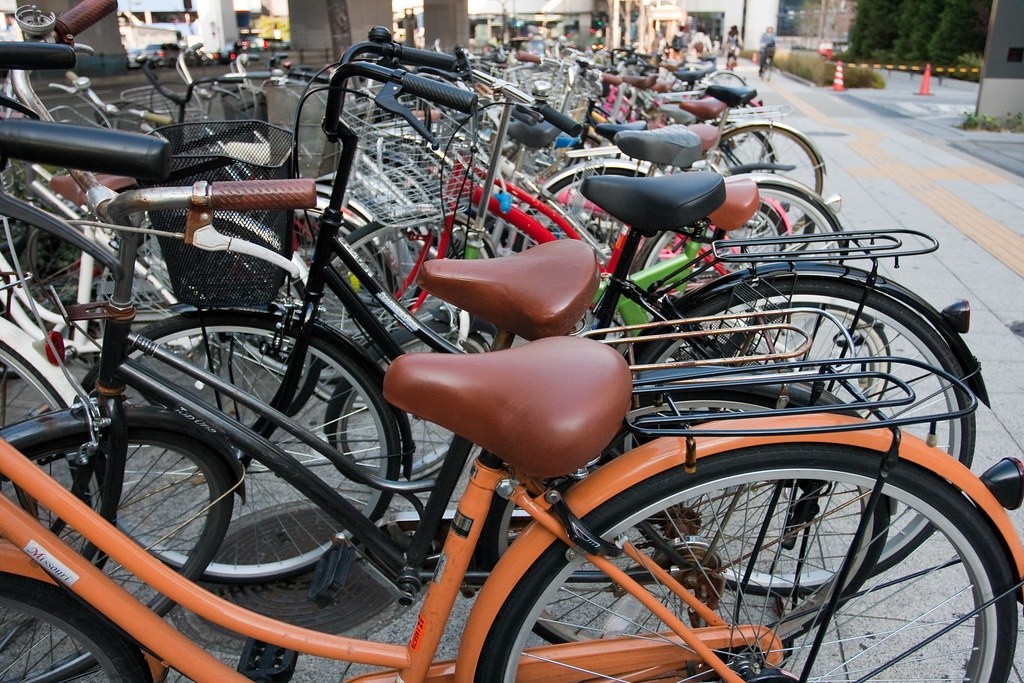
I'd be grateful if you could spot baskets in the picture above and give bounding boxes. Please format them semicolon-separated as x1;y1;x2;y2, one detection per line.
504;62;592;131
137;116;298;305
119;82;205;152
338;93;470;230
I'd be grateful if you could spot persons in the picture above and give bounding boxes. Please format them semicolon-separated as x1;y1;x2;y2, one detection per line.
758;25;777;77
725;24;743;66
646;15;725;69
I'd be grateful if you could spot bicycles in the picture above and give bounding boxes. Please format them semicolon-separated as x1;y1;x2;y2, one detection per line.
0;0;994;683
0;34;1023;682
